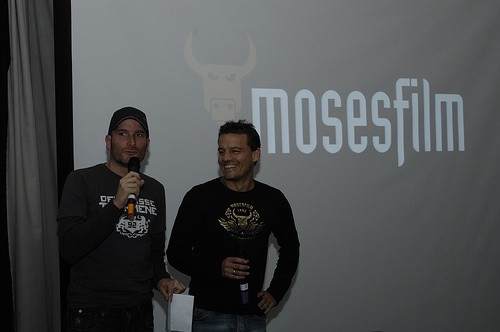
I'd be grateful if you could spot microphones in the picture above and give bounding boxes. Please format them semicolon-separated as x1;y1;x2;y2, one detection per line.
128;157;140;216
238;248;249;304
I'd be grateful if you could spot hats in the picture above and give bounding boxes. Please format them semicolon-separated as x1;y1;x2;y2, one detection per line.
109;107;149;137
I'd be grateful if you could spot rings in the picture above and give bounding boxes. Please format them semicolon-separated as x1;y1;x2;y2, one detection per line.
233;270;236;274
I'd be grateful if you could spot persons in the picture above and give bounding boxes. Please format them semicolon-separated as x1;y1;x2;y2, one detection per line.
55;106;186;332
166;118;300;332
